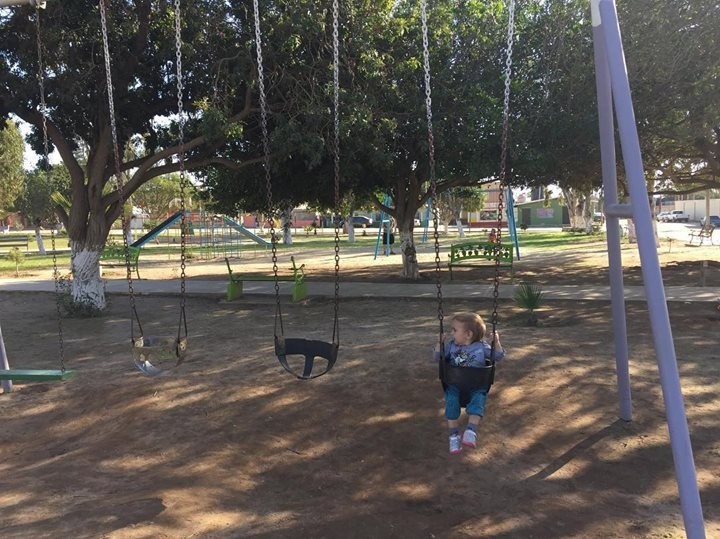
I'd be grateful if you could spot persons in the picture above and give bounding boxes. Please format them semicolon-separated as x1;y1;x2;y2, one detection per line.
430;313;505;456
488;229;499;245
382;214;397;256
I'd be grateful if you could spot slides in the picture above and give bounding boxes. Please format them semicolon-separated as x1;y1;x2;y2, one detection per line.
131;211;183;247
223;217;278;245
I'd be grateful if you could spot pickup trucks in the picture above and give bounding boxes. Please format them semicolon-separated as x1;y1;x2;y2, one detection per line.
667;210;689;223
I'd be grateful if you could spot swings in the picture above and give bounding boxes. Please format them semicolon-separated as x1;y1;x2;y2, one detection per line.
420;1;515;407
252;0;341;379
0;0;72;380
99;0;189;374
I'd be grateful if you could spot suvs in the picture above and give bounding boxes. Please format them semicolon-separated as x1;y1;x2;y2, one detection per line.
339;215;373;228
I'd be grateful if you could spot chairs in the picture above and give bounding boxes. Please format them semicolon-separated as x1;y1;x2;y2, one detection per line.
688;223;717;247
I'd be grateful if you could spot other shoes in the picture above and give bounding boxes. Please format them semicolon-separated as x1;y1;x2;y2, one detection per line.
383;251;387;255
390;251;396;254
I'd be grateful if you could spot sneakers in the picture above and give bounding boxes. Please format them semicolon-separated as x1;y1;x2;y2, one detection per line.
448;435;462;454
462;430;477;449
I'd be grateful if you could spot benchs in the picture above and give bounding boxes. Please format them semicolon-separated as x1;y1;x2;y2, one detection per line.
97;242;145;283
224;256;308;303
448;242;514;283
0;234;30;252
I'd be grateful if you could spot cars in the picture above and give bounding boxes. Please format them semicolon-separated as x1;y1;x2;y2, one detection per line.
656;211;671;222
699;216;720;228
